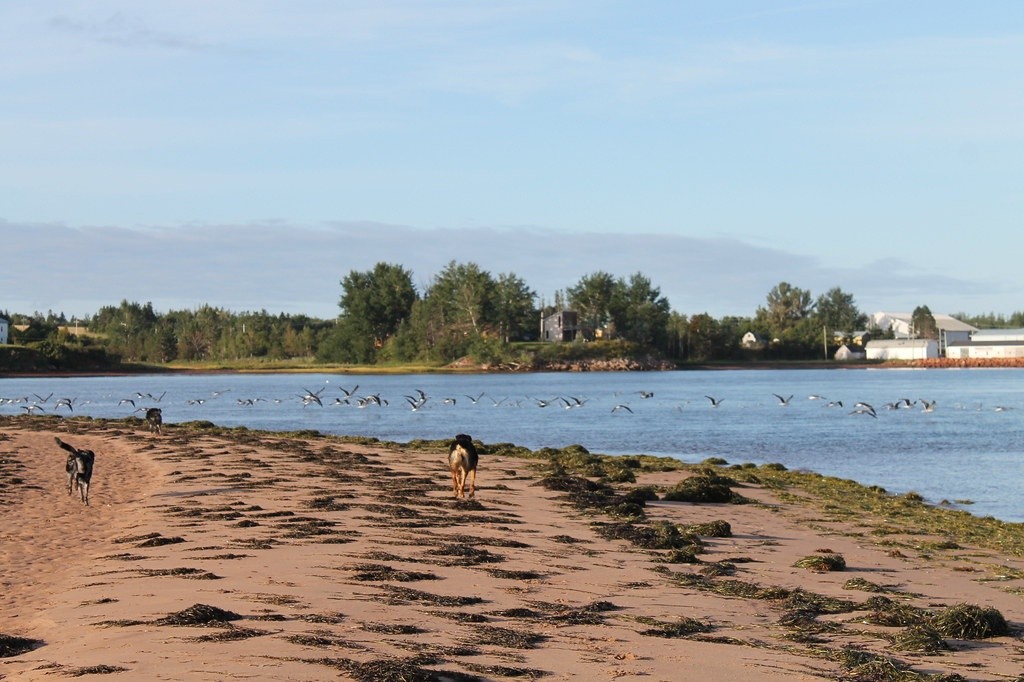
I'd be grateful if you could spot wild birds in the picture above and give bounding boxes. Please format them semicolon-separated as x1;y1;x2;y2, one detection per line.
0;385;1011;418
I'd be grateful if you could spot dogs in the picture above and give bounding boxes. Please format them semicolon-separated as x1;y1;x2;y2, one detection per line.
146;408;162;435
54;436;94;506
450;434;479;498
